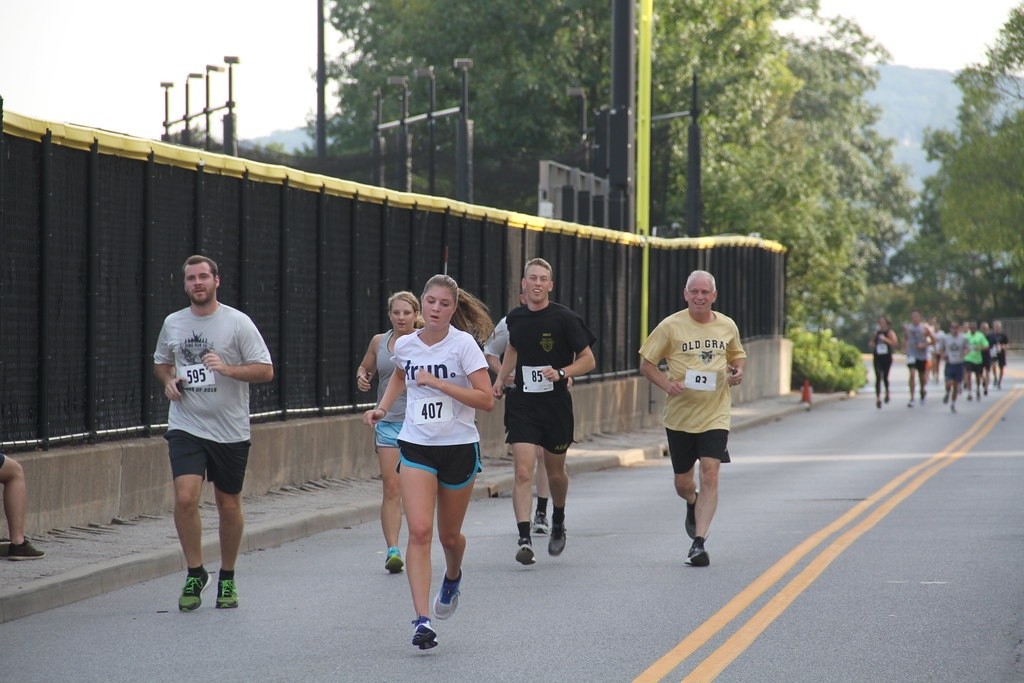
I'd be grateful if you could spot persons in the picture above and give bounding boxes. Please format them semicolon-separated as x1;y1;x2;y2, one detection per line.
356;291;425;573
493;258;597;565
638;269;747;566
153;256;274;611
484;288;573;533
0;453;45;560
901;310;1009;413
363;274;495;650
869;314;898;408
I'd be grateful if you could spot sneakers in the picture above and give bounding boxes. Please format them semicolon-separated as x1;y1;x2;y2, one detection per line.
434;570;463;619
534;514;549;534
178;571;208;612
685;493;699;540
386;549;403;574
215;580;239;608
685;544;710;567
8;540;46;560
412;619;438;649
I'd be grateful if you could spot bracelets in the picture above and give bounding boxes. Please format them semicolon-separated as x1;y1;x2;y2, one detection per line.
356;376;360;385
378;408;387;416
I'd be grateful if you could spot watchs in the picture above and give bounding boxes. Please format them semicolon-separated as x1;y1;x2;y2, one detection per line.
557;368;565;380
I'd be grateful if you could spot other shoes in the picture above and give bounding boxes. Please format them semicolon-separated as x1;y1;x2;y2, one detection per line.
516;543;535;564
549;523;566;555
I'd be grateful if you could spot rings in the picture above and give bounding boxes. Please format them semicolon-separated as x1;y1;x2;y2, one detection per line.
737;381;739;384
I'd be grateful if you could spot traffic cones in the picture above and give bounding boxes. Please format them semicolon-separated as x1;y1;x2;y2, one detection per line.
801;379;813;403
